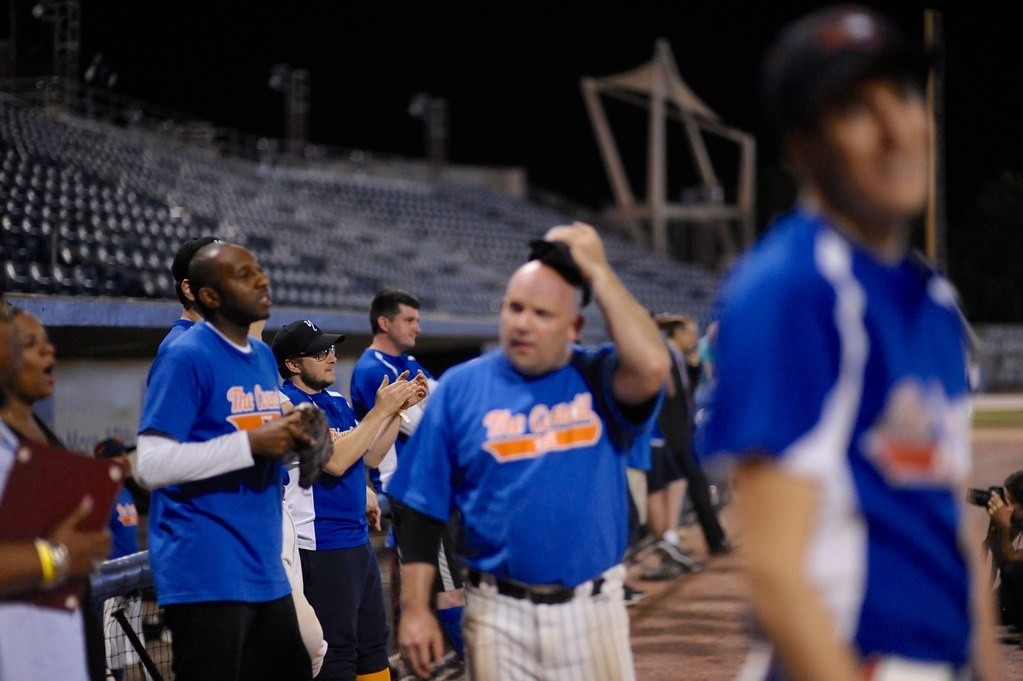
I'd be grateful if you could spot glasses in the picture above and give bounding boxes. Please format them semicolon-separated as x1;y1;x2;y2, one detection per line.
315;343;336;362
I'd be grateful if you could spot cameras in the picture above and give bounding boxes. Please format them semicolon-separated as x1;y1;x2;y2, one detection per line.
966;487;1006;509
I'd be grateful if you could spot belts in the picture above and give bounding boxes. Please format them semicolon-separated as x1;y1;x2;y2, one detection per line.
465;571;606;606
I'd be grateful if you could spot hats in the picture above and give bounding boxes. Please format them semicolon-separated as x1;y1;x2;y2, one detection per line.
273;319;345;360
92;438;140;461
768;11;938;113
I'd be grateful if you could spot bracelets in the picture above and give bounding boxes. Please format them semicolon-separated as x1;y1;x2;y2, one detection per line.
32;537;69;589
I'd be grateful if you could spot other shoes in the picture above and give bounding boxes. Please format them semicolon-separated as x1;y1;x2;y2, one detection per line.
641;555;684;581
998;630;1023;648
713;539;743;556
624;584;650;605
655;538;703;573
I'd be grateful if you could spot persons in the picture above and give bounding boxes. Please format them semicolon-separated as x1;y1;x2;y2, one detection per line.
95;436;147;681
568;314;734;607
695;11;1000;681
272;320;428;681
159;238;328;681
348;291;466;653
132;240;333;681
0;296;116;681
987;470;1023;628
0;308;105;681
385;220;672;681
